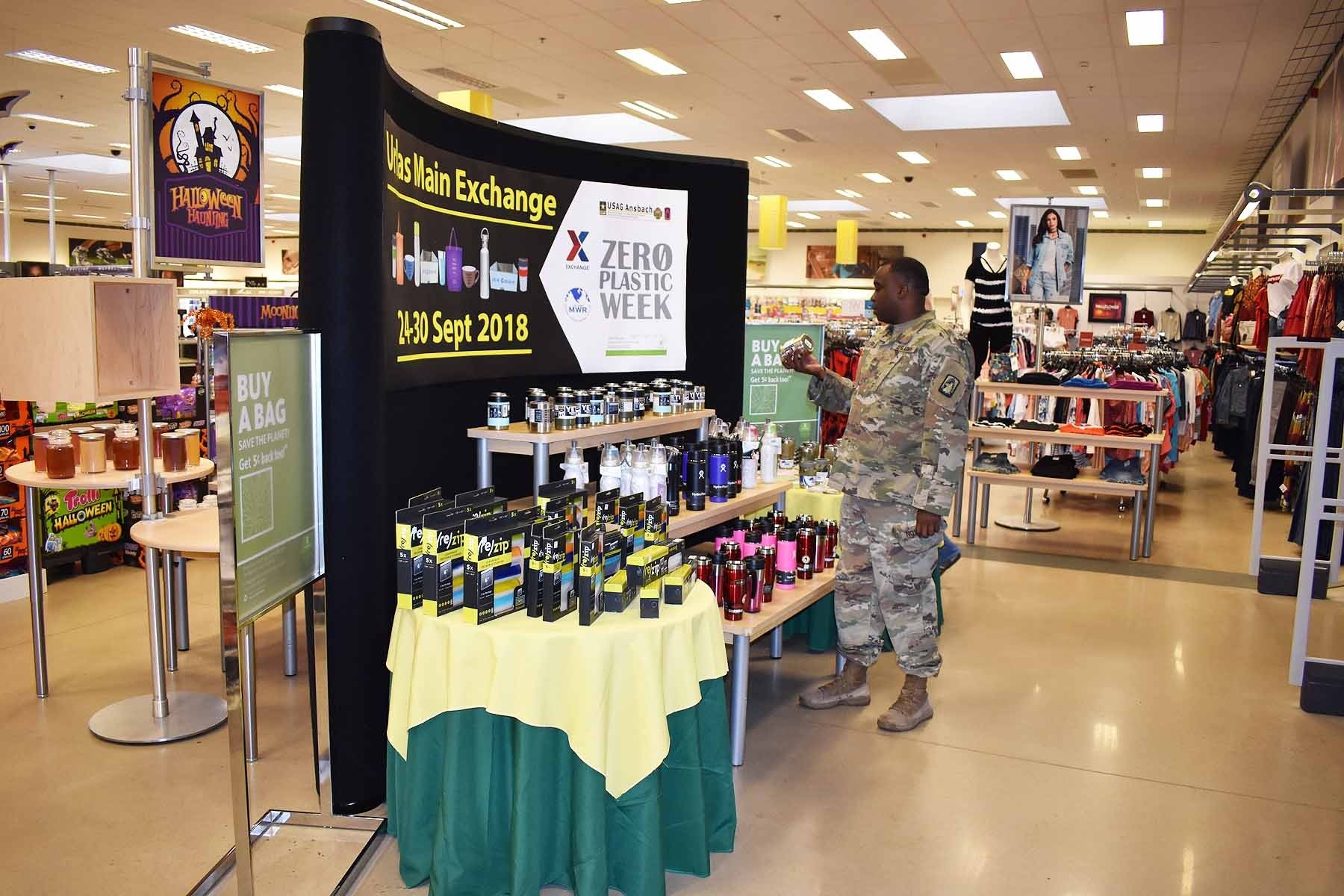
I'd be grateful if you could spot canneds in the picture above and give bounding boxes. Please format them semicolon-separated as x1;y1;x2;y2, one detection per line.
525;379;705;433
779;333;814;362
32;422;200;479
487;392;510;430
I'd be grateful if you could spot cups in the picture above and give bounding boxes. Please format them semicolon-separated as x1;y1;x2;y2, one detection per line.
462;265;480;288
404;254;414;281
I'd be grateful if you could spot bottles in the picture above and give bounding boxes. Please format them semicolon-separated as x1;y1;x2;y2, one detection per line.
558;414;847;622
31;421;202;479
480;227;490;299
178;493;218;511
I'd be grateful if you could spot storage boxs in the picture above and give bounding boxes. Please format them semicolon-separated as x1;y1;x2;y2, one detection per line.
396;478;697;627
0;385;209;577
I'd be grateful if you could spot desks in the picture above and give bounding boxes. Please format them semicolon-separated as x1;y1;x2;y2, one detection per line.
5;457;297;747
386;578;737;896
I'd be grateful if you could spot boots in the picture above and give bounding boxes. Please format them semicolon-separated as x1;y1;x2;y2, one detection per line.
877;677;934;732
798;659;873;709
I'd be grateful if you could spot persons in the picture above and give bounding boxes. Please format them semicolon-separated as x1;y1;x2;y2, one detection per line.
1221;276;1244;320
1022;209;1073;302
1239;268;1267;323
962;241;1014;381
1269;249;1302;340
779;257;975;732
1204;291;1223;339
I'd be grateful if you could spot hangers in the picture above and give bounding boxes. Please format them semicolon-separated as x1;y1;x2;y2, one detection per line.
1303;251;1344;279
1043;346;1187;376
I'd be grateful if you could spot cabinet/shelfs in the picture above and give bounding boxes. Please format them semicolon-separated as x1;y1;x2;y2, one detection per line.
468;409;846;766
953;377;1168;560
